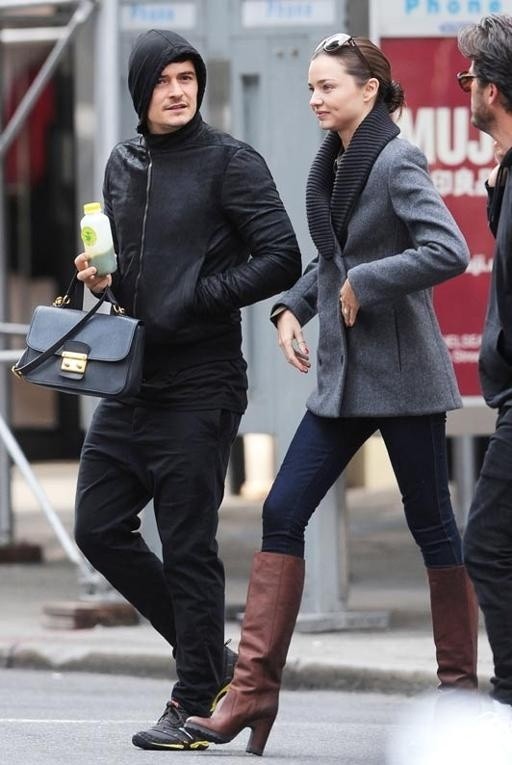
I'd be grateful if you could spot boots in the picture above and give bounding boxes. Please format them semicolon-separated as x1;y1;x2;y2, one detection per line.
183;552;305;754
429;567;480;690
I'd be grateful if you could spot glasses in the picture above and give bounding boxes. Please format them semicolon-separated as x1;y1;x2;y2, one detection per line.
457;70;486;92
315;32;378;82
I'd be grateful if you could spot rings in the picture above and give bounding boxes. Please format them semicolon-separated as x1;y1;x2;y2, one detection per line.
344;309;349;316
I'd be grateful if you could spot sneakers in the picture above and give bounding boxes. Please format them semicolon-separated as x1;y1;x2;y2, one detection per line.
131;639;237;751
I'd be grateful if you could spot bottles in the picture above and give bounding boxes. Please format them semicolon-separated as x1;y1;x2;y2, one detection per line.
79;201;119;277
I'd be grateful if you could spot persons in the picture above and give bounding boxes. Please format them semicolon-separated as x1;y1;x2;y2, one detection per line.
453;13;511;713
184;29;483;759
73;25;304;753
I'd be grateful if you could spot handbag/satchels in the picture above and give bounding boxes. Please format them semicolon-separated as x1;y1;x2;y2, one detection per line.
13;302;148;402
